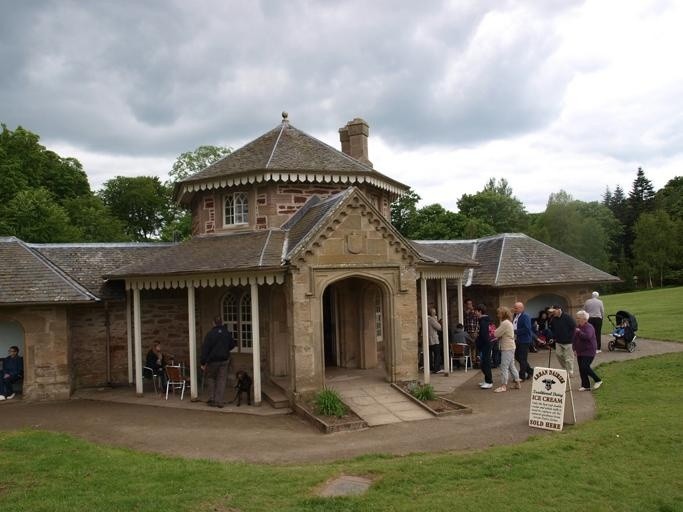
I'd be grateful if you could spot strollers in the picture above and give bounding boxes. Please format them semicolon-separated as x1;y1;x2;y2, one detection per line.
605;310;638;353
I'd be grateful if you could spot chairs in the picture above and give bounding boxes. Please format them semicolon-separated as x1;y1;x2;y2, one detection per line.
143;366;160;394
165;365;190;400
450;343;472;373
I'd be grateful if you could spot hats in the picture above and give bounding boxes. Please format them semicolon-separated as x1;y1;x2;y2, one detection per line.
548;304;562;312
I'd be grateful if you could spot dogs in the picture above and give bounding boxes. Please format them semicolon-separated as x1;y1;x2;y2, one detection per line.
233;369;252;407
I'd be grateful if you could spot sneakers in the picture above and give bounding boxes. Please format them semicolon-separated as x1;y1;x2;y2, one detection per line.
596;349;601;354
493;386;506;392
527;368;533;379
578;387;591;391
592;381;602;390
0;393;16;401
478;381;493;388
510;384;521;388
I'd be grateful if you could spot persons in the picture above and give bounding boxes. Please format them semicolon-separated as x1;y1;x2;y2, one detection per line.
427;298;553;393
572;310;604;392
548;303;576;378
145;340;171;394
0;346;23;401
200;317;234;407
582;292;605;353
612;319;629;339
